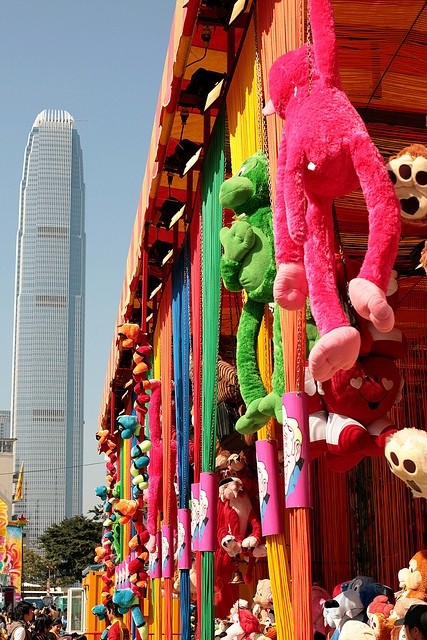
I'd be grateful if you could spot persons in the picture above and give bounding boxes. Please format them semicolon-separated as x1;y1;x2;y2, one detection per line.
104;598;131;640
0;600;71;640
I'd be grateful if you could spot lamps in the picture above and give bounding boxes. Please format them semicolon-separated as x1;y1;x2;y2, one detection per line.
156;195;187;231
148;240;173;269
187;66;226;113
139;274;162;301
136;305;153;322
114;378;132;436
166;138;203;177
202;2;247;28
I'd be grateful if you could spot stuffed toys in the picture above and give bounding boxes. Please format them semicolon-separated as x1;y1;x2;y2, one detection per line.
381;141;427;505
116;322;156;414
218;149;320;436
92;545;114;603
113;411;150;499
269;0;404;381
309;329;402;476
213;444;269;561
112;588;148;639
93;605;112;639
94;430;120;485
110;494;151;602
94;485;114;545
312;549;427;639
212;578;277;639
